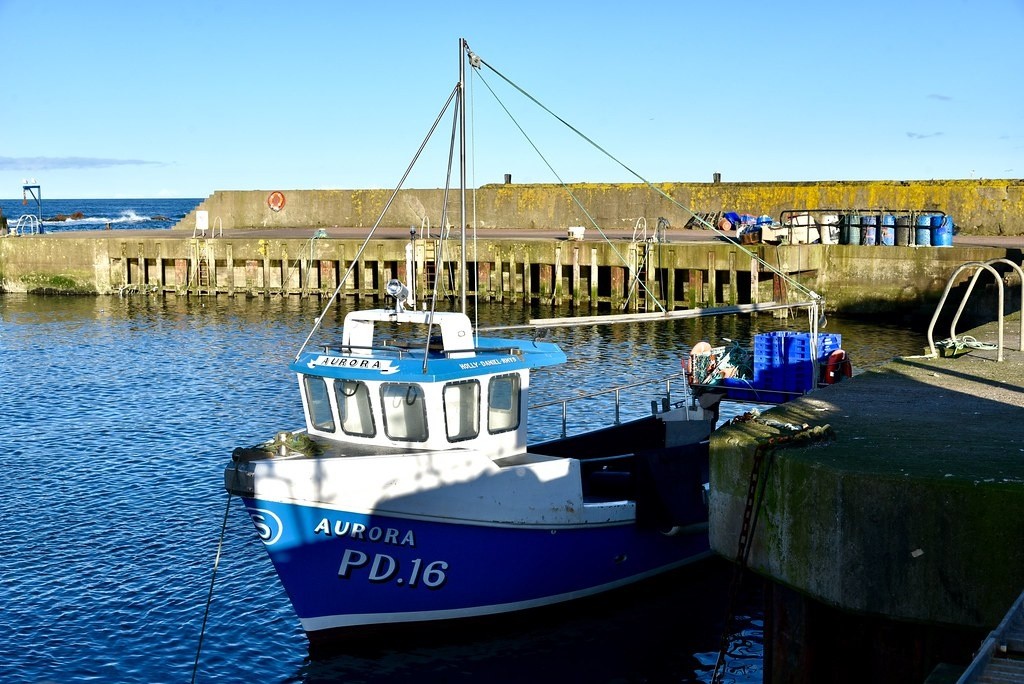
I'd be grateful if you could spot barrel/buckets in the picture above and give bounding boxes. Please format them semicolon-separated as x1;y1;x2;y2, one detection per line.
845;215;861;246
820;214;840;244
757;216;773;226
895;217;916;246
916;215;932;246
932;216;952;247
878;215;895;245
719;218;732;231
861;216;878;245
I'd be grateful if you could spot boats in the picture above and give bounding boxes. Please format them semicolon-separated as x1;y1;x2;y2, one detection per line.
220;37;856;656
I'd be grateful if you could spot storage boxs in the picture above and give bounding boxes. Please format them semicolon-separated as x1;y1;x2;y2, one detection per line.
725;332;841;403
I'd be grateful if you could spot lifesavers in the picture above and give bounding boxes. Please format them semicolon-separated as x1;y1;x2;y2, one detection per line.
687;342;712;385
268;192;283;208
825;350;852;382
